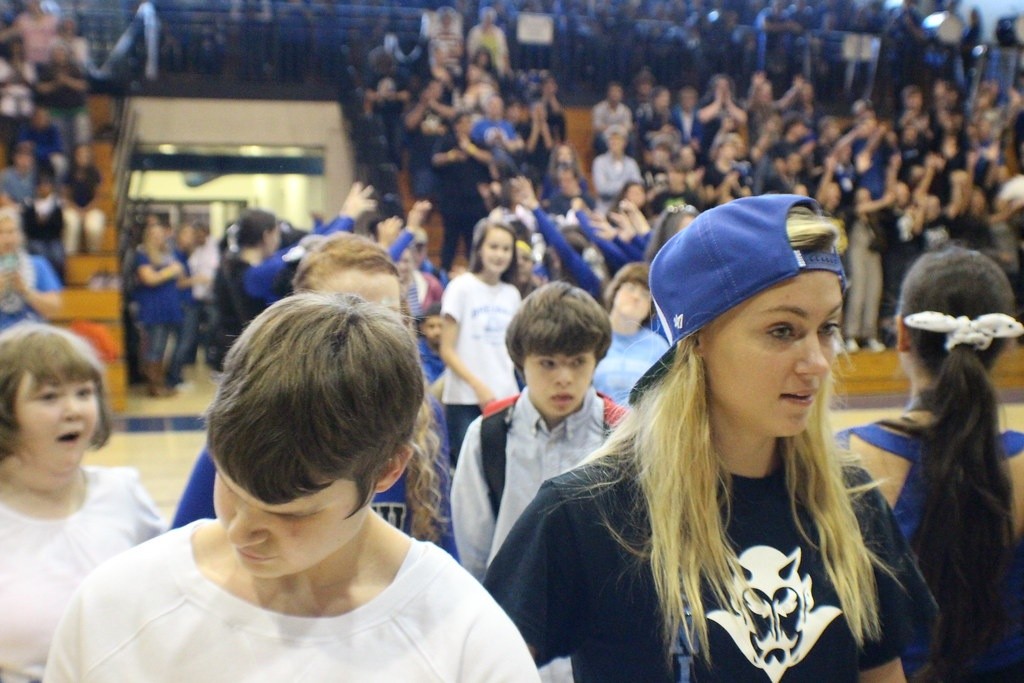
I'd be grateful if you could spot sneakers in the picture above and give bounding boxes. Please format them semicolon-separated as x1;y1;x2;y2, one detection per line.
869;339;885;352
843;338;859;354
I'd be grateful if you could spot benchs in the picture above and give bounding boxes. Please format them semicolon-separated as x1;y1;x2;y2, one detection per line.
0;0;1023;418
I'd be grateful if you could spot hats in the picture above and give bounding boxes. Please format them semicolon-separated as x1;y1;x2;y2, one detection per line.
627;193;849;405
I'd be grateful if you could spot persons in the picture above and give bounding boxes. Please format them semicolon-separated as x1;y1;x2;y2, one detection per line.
437;217;524;467
162;230;463;566
0;319;170;683
446;280;632;683
832;246;1024;683
0;0;1023;404
41;289;542;683
479;194;943;683
